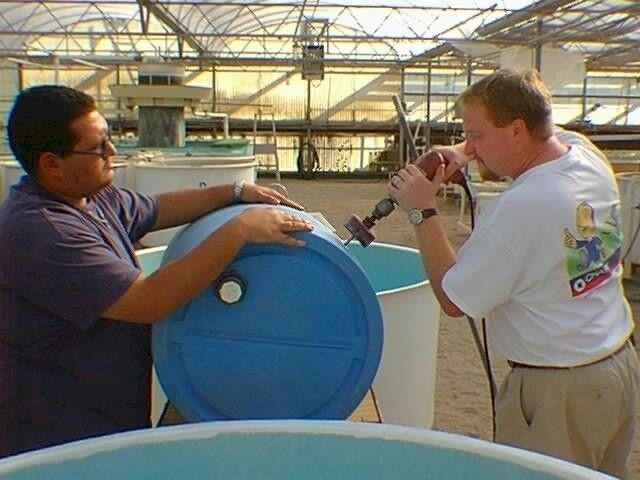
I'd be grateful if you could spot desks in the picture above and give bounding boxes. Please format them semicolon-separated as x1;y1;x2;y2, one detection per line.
157;386;383;426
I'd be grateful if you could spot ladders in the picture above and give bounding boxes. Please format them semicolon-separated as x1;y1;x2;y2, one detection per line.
250;112;281;182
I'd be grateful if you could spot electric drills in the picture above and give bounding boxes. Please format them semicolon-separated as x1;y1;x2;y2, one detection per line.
342;150;472;253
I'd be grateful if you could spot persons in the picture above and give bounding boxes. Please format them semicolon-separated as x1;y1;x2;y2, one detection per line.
0;85;314;459
386;69;640;480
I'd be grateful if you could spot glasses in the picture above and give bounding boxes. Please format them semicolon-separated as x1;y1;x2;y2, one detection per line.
73;124;114;159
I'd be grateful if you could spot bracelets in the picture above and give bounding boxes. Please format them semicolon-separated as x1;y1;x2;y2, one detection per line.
233;181;245;202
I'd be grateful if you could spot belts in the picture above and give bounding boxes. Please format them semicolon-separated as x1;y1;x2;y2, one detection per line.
507;332;633;371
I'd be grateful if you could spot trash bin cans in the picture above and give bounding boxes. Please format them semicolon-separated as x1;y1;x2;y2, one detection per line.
615;172;639;265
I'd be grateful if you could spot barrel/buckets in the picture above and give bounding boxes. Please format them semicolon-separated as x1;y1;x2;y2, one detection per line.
151;204;384;422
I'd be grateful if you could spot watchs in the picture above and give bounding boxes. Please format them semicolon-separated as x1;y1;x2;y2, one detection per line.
408;208;437;225
